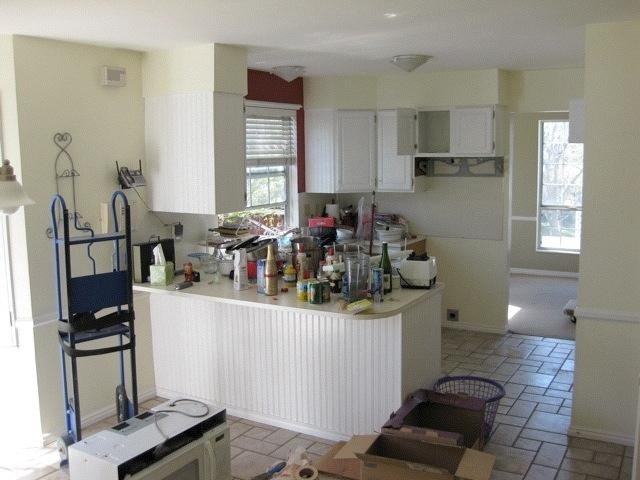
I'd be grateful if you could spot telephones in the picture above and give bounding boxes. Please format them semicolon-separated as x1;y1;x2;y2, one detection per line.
116;160;147;189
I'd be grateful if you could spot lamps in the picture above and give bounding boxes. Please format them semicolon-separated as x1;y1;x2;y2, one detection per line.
0;159;36;216
390;54;432;72
272;66;305;83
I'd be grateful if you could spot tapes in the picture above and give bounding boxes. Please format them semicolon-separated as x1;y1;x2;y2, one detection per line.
295;465;318;480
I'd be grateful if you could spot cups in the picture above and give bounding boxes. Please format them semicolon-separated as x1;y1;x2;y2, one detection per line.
345;258;359;302
357;254;371;295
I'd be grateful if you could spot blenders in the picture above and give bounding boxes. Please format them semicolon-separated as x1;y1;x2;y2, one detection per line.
400;252;438;288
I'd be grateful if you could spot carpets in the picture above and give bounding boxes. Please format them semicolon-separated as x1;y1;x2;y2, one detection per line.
508;274;579;341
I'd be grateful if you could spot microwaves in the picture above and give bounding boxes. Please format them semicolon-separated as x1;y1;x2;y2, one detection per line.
67;397;232;480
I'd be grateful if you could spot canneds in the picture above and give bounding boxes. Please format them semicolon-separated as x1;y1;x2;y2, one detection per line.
373;267;384;302
294;242;331;304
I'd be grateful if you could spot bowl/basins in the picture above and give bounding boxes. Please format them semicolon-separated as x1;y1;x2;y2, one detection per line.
337;228;353;240
201;256;218;274
219;260;234;276
375;226;404;241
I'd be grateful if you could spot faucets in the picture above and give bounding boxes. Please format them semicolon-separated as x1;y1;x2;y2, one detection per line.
278;228;302;238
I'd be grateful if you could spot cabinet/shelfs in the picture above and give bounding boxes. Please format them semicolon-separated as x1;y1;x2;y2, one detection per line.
396;106;493;155
305;110;375;194
375;110;415;193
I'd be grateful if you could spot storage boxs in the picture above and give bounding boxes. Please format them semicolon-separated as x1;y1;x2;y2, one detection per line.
311;389;496;480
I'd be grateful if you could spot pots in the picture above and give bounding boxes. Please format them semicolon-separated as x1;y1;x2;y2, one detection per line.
289;236;321;252
286;251;322;270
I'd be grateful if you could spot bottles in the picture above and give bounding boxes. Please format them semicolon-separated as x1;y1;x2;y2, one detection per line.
265;244;278;296
379;242;393;293
330;269;342;293
326;247;336;265
342;243;351;262
